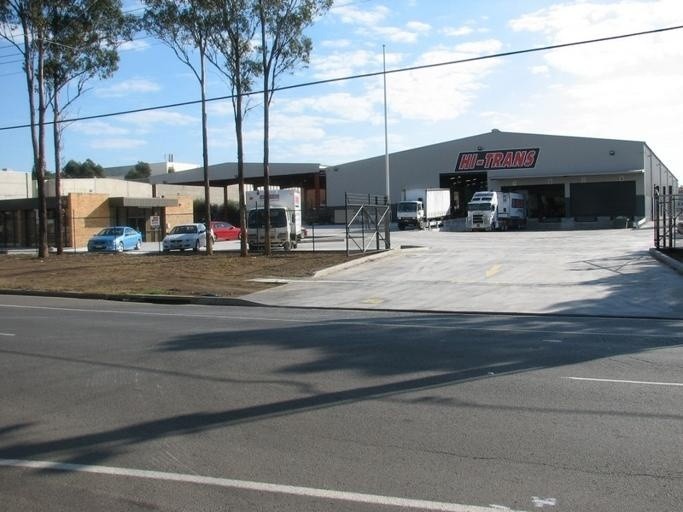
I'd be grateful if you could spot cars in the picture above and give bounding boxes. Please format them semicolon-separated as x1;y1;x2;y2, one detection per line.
162;221;241;253
87;226;142;252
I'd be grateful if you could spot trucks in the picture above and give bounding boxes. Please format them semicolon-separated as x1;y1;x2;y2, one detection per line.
396;188;451;230
245;189;302;249
466;190;527;233
